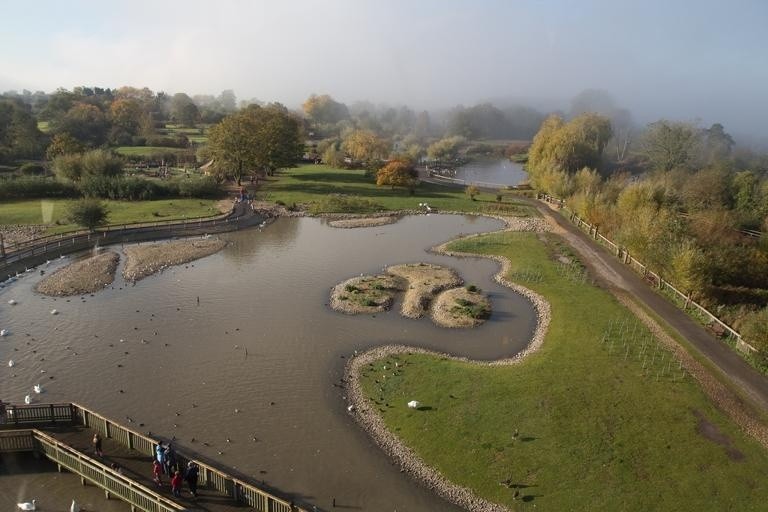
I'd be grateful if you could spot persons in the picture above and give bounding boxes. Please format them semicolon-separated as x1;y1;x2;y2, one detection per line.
151;440;200;497
92;433;104;456
0;399;7;429
239;185;247;203
